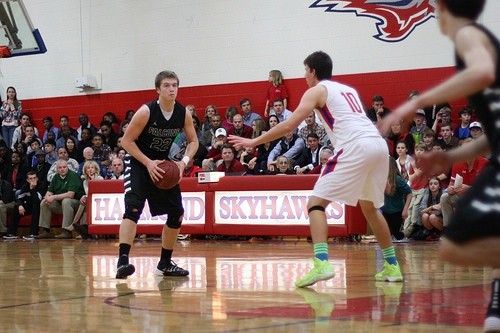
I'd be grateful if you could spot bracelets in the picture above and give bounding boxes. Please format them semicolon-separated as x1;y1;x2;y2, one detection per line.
180;156;190;167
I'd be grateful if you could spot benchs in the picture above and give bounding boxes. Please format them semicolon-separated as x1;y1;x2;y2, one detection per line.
6;208;63;227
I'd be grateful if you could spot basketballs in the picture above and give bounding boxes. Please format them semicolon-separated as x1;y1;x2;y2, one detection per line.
154;160;181;190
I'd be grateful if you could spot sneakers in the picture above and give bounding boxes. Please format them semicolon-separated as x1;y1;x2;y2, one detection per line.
116;255;135;279
295;256;336;287
375;260;403;282
157;260;188;277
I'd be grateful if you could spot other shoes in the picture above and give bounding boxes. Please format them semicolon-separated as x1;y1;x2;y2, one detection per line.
413;229;424;240
3;232;18;238
64;224;76;231
23;233;34;240
393;232;404;240
417;228;431;240
34;231;52;239
426;232;445;240
482;278;500;332
76;234;82;239
55;229;73;238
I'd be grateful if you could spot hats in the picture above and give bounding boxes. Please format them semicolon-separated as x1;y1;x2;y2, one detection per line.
469;121;482;130
415;108;425;117
34;149;47;157
215;128;227;137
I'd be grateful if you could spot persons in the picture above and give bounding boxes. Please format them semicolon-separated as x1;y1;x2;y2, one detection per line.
115;72;199;279
376;0;500;333
0;70;494;240
227;52;403;288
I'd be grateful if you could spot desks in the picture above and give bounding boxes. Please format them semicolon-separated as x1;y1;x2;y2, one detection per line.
86;174;367;244
